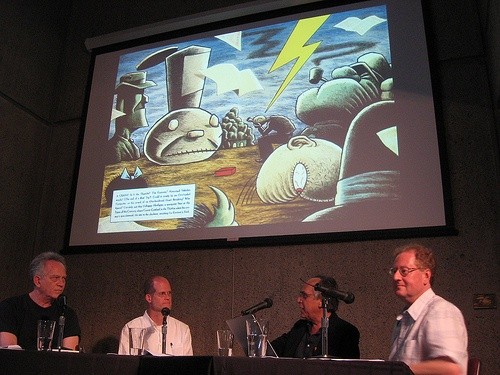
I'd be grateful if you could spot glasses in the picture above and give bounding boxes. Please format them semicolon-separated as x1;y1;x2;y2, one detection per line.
389;266;424;276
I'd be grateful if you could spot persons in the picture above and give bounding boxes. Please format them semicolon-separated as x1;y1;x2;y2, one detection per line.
0;252;82;351
117;275;194;357
267;275;360;359
388;243;469;375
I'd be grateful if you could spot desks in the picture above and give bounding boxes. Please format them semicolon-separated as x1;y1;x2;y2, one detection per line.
1;348;417;375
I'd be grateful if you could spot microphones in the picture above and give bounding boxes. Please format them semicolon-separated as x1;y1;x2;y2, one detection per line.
240;298;274;316
160;307;170;334
57;292;67;324
313;283;355;305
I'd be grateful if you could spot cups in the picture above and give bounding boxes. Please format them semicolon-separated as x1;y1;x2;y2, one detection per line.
37;320;55;350
216;330;235;356
245;319;269;357
